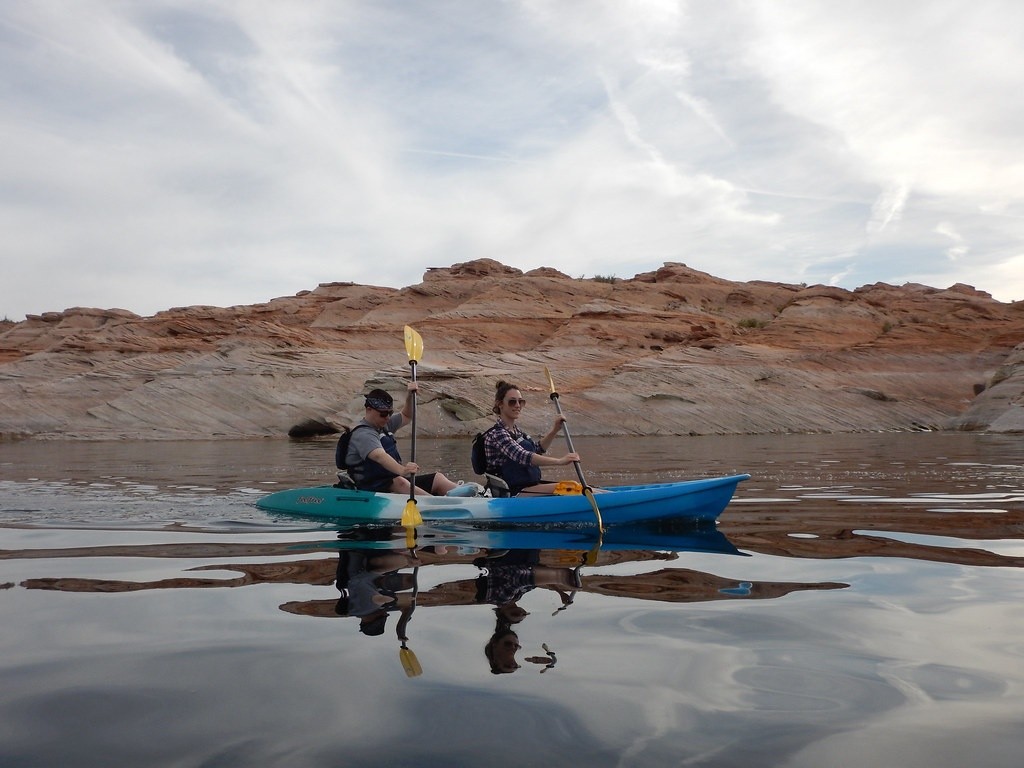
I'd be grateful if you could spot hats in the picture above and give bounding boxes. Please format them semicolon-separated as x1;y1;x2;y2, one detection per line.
358;614;391;635
365;389;393;411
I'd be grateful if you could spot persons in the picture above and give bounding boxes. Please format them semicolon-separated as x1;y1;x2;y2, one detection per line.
484;380;614;498
347;382;461;496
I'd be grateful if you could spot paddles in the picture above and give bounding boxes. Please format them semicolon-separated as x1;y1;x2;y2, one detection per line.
399;642;425;679
401;324;424;525
551;591;578;618
542;364;603;534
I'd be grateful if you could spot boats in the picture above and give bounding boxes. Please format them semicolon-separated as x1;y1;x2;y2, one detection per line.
255;473;752;525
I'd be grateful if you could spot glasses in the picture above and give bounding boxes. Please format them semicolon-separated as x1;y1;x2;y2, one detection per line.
372;408;394;418
499;400;526;408
